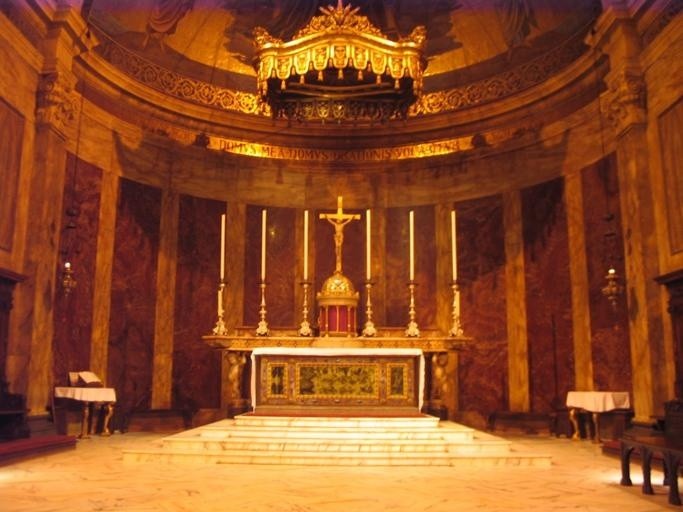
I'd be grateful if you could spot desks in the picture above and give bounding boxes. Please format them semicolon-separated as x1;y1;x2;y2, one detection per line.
55;387;117;440
566;392;631;443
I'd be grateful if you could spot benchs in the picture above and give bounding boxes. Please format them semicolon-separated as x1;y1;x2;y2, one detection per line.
485;412;560;439
0;394;31;437
620;400;683;505
120;409;195;434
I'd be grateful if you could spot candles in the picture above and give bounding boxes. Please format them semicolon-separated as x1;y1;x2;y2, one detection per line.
262;210;267;282
220;215;225;279
367;210;371;280
451;211;458;281
408;211;415;283
304;211;308;279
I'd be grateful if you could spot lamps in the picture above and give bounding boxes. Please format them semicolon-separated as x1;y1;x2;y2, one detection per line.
63;262;76;293
602;269;626;305
